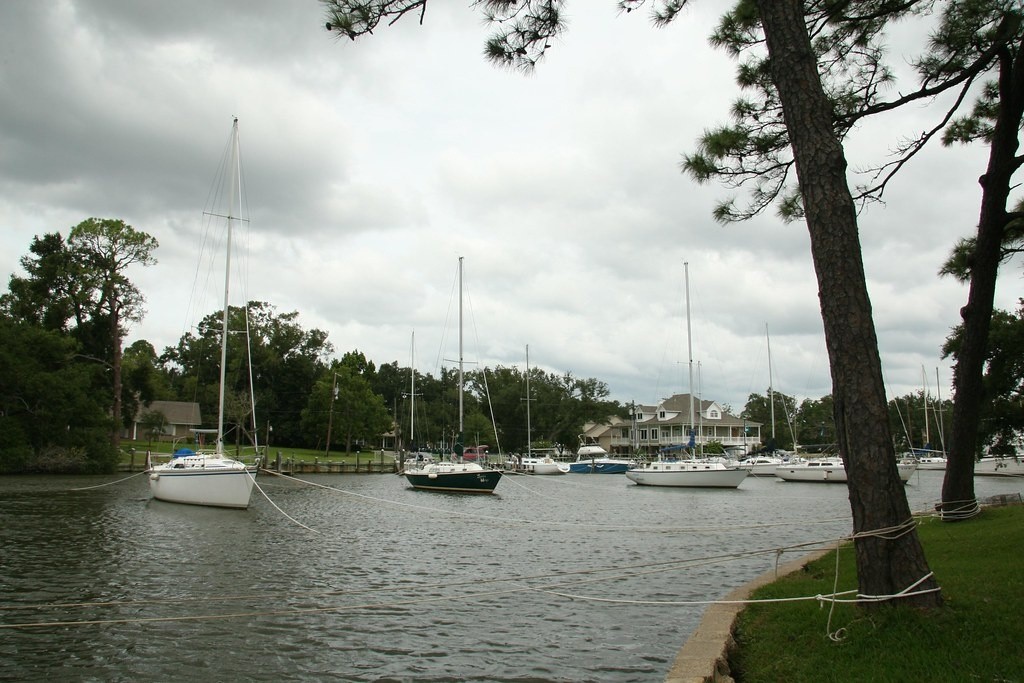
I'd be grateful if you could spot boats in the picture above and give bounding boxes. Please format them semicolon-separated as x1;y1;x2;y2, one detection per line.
973;454;1024;478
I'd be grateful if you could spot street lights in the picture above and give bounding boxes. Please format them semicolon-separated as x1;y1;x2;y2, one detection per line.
325;372;343;457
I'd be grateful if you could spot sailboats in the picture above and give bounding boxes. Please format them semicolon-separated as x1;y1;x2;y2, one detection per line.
144;113;263;512
733;321;949;484
468;342;640;477
398;259;505;495
624;260;751;489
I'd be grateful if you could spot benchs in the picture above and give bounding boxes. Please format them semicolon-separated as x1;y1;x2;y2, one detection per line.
198;449;217;455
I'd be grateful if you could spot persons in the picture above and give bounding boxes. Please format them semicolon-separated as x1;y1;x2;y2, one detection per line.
439;450;443;461
418;453;423;460
509;452;512;461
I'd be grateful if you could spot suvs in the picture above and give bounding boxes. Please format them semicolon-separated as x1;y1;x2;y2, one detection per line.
451;444;489;462
